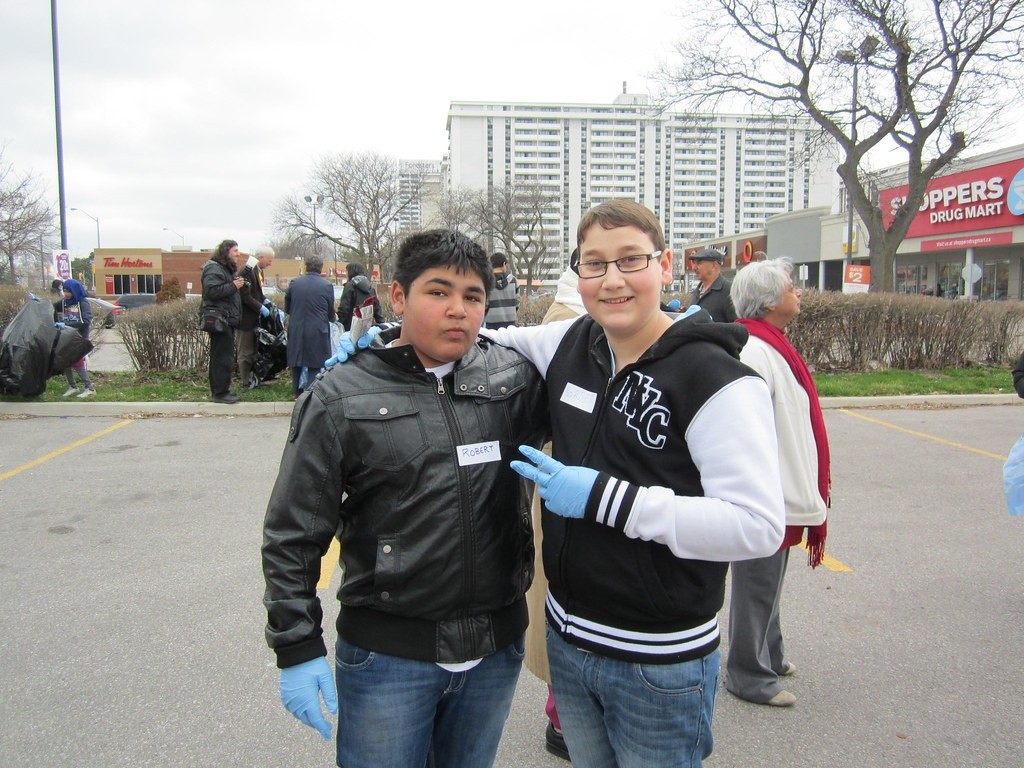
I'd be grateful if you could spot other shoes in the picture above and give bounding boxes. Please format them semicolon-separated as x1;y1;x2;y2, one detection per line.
76;389;96;398
766;690;795;705
213;393;239;403
212;391;237;399
783;662;795;676
62;387;79;397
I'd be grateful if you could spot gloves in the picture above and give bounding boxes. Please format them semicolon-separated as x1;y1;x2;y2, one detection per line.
324;325;382;366
260;305;270;317
510;445;599;518
278;657;336;742
264;300;270;304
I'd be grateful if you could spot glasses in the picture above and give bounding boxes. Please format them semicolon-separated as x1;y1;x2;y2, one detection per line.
572;250;663;278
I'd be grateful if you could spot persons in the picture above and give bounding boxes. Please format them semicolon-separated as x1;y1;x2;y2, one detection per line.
722;258;831;705
236;247;275;390
685;249;739;324
284;256;336;401
477;250;520;337
1011;350;1024;399
198;240;244;404
914;282;958;299
337;262;382;336
325;199;788;768
28;280;97;397
525;244;602;761
51;280;68;300
262;230;680;768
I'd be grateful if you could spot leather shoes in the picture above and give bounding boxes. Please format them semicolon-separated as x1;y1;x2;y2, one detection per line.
546;721;571;763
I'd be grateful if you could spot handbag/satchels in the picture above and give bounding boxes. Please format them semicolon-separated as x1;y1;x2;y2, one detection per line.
200;307;229;332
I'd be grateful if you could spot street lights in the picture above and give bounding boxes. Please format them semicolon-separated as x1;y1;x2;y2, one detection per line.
69;208;100;248
305;194;327;252
330;237;343;286
161;228;185;250
835;33;880;264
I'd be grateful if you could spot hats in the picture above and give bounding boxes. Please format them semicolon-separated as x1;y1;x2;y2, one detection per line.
689;248;721;263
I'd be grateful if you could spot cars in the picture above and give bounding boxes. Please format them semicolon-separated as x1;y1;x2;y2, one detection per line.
110;292;158;312
262;287;285;297
331;284;344;303
84;297;127;329
